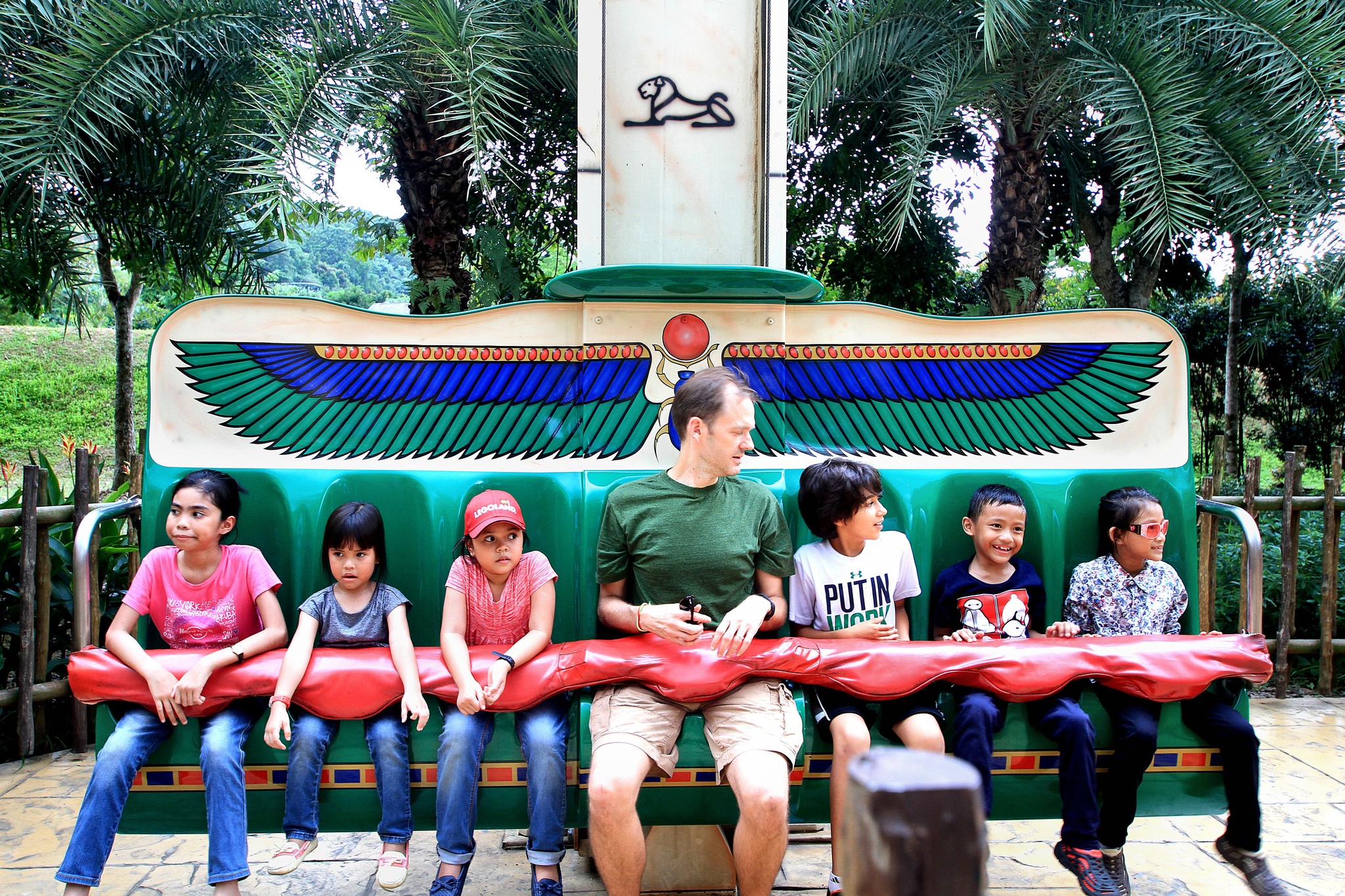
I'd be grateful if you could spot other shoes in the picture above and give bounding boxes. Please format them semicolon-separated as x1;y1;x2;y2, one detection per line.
530;863;563;896
429;862;470;896
377;841;409;888
268;837;318;875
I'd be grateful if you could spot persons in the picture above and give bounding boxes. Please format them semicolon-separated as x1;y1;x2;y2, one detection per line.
930;482;1120;896
264;499;431;893
787;457;948;896
594;366;804;896
55;467;289;896
437;488;571;895
1061;486;1293;896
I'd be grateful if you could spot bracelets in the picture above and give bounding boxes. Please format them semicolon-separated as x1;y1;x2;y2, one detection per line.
756;593;775;622
491;651;515;675
636;600;651;632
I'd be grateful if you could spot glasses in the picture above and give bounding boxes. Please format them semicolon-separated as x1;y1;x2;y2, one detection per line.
1126;520;1169;539
679;594;697;625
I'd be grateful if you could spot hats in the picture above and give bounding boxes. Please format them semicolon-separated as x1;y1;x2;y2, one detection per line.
463;489;526;539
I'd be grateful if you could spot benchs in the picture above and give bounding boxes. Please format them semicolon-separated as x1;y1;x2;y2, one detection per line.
74;464;1260;820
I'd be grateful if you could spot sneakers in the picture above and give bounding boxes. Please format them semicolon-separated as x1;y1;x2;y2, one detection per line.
1215;836;1287;895
1054;839;1122;896
1101;846;1131;896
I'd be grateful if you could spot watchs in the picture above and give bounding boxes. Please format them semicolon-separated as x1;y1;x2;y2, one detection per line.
227;644;245;664
267;695;291;710
